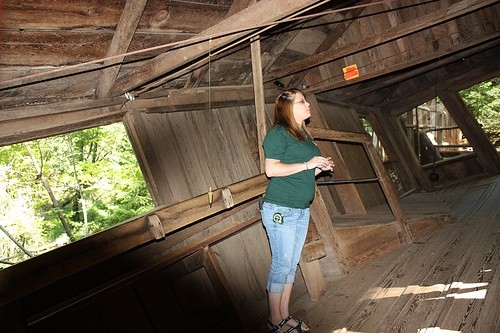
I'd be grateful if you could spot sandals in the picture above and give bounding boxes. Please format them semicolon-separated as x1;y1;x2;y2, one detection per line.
265;315;311;333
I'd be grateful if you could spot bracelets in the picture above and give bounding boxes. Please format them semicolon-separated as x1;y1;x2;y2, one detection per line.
304;162;307;171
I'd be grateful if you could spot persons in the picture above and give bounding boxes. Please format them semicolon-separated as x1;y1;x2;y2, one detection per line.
259;87;334;333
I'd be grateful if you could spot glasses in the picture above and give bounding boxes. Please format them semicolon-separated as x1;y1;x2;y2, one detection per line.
294;99;308;104
